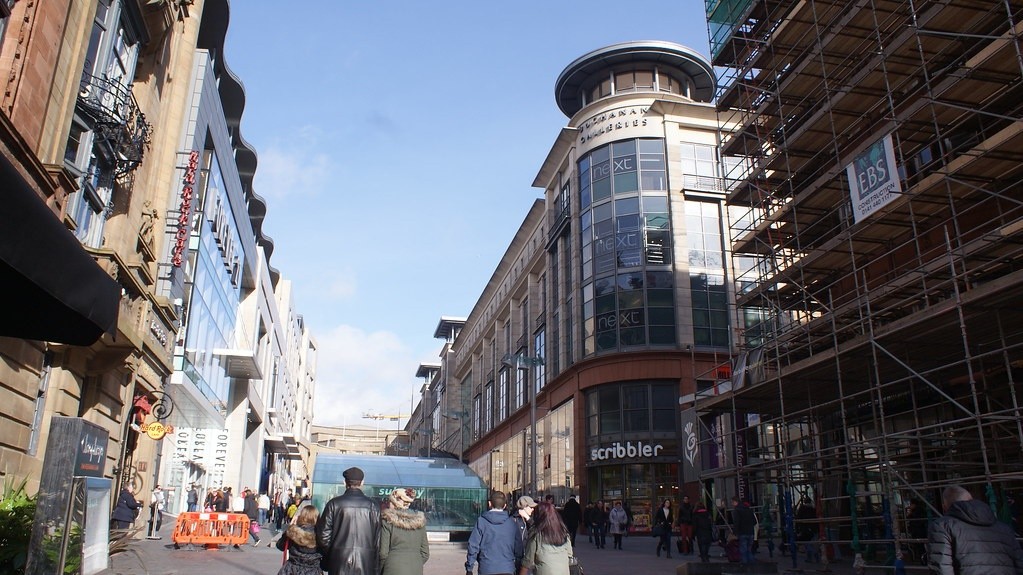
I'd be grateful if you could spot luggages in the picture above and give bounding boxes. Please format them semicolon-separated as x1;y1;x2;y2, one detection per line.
677;534;690;553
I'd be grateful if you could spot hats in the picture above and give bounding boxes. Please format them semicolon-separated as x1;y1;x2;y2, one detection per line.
389;488;416;509
588;501;593;504
244;489;251;493
516;496;538;509
343;468;364;480
570;494;576;498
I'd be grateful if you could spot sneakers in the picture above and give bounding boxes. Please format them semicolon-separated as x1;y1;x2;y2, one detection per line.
275;529;283;533
254;540;261;547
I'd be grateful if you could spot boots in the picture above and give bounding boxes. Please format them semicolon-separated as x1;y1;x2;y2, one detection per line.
614;542;622;550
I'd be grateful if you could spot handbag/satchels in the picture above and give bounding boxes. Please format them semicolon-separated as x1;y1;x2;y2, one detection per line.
652;526;666;537
568;557;584;575
619;523;625;530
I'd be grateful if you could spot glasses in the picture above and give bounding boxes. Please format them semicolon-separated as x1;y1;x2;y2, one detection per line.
158;488;162;491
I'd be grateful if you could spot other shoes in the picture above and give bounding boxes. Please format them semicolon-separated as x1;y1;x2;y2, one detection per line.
657;547;660;556
589;540;592;543
597;546;604;549
667;556;672;559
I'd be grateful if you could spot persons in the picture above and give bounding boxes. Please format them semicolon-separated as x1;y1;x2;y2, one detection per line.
315;467;378;575
926;485;1023;575
464;491;522;575
651;498;674;558
510;496;537;575
112;482;142;544
714;494;760;563
520;502;583;575
186;481;311;549
675;495;694;556
584;498;631;550
533;496;540;503
692;502;713;563
998;485;1023;549
906;498;946;565
149;485;165;536
797;497;819;563
561;495;580;548
378;487;430;575
547;495;554;504
276;505;324;575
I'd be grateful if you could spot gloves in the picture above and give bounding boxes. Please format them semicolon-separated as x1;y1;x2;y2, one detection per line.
466;571;473;575
